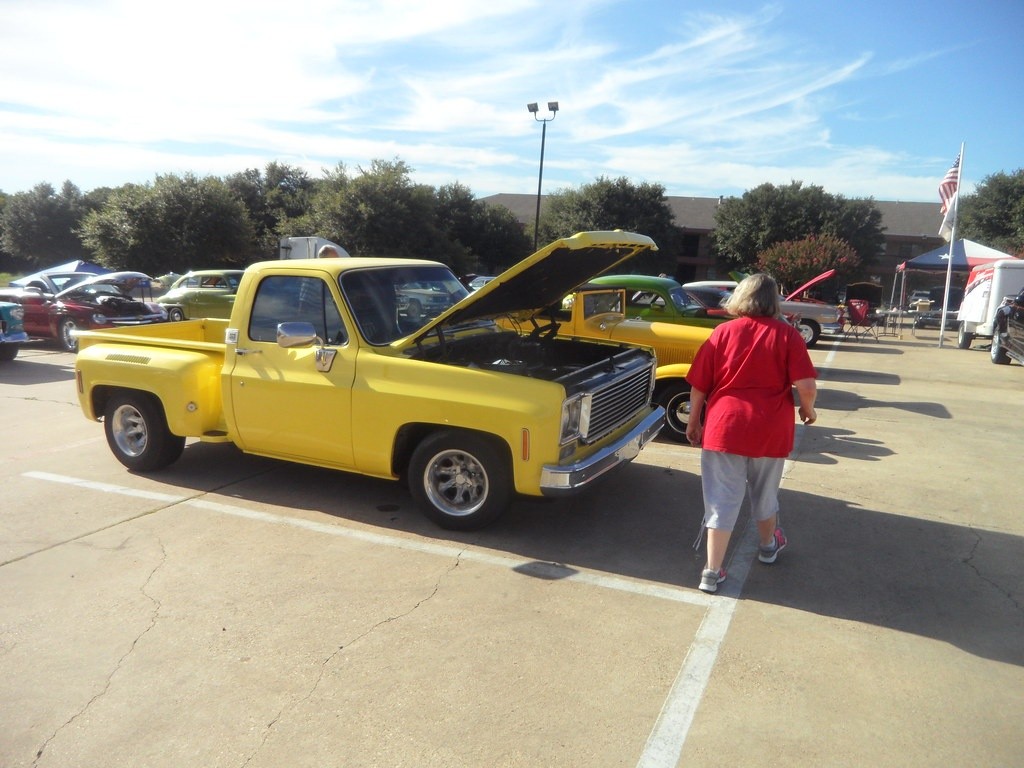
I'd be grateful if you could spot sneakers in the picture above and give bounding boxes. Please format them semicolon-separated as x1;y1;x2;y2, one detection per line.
698;562;726;592
758;528;787;563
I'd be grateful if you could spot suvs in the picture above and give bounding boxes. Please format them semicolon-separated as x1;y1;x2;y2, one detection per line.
913;287;963;332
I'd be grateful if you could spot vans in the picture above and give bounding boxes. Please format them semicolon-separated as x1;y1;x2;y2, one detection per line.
956;258;1024;353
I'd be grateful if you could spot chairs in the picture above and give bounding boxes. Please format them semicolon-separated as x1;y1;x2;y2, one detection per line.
841;300;885;343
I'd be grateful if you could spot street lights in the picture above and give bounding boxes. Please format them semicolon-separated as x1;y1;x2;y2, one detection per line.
527;100;559;258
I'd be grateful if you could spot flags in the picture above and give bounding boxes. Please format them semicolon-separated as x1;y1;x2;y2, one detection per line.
938;191;957;241
938;153;960;215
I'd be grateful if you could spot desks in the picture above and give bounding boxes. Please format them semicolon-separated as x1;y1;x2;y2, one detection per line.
876;309;962;338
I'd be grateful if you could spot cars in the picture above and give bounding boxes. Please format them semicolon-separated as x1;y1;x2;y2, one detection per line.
906;290;932;310
679;268;843;350
151;269;245;321
387;272;494;327
0;270;169;354
989;287;1024;368
0;302;30;363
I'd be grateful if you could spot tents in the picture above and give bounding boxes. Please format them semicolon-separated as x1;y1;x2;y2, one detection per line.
885;239;1021;336
9;260;153;303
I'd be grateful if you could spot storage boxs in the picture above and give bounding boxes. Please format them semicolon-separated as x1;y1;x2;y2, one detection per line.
910;299;935;311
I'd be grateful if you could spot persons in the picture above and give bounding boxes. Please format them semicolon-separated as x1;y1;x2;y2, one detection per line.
685;272;818;591
659;273;666;278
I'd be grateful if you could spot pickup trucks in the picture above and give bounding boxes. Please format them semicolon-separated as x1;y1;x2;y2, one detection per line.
486;273;740;447
73;228;668;536
